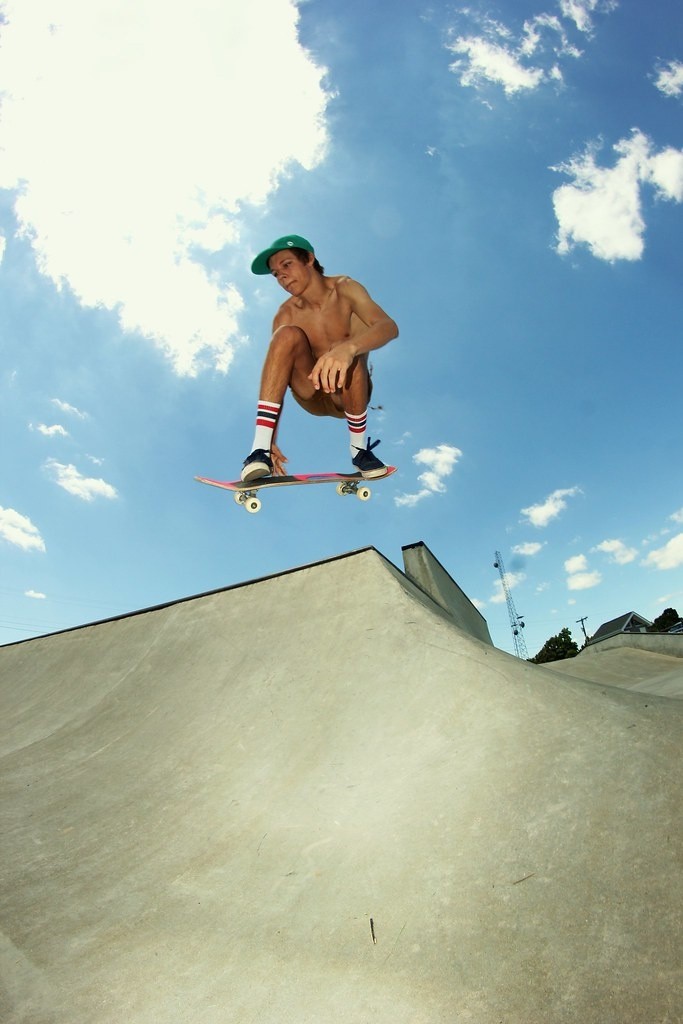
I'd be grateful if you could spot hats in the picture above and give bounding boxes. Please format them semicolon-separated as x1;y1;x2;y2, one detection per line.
249;235;315;276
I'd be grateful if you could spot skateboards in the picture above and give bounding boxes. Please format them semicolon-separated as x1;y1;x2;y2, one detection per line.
196;465;399;513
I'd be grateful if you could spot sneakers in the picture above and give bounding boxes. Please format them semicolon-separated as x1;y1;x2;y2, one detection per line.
239;448;273;482
351;436;387;479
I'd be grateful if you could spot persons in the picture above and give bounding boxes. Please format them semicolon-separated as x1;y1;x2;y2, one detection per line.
241;235;398;482
